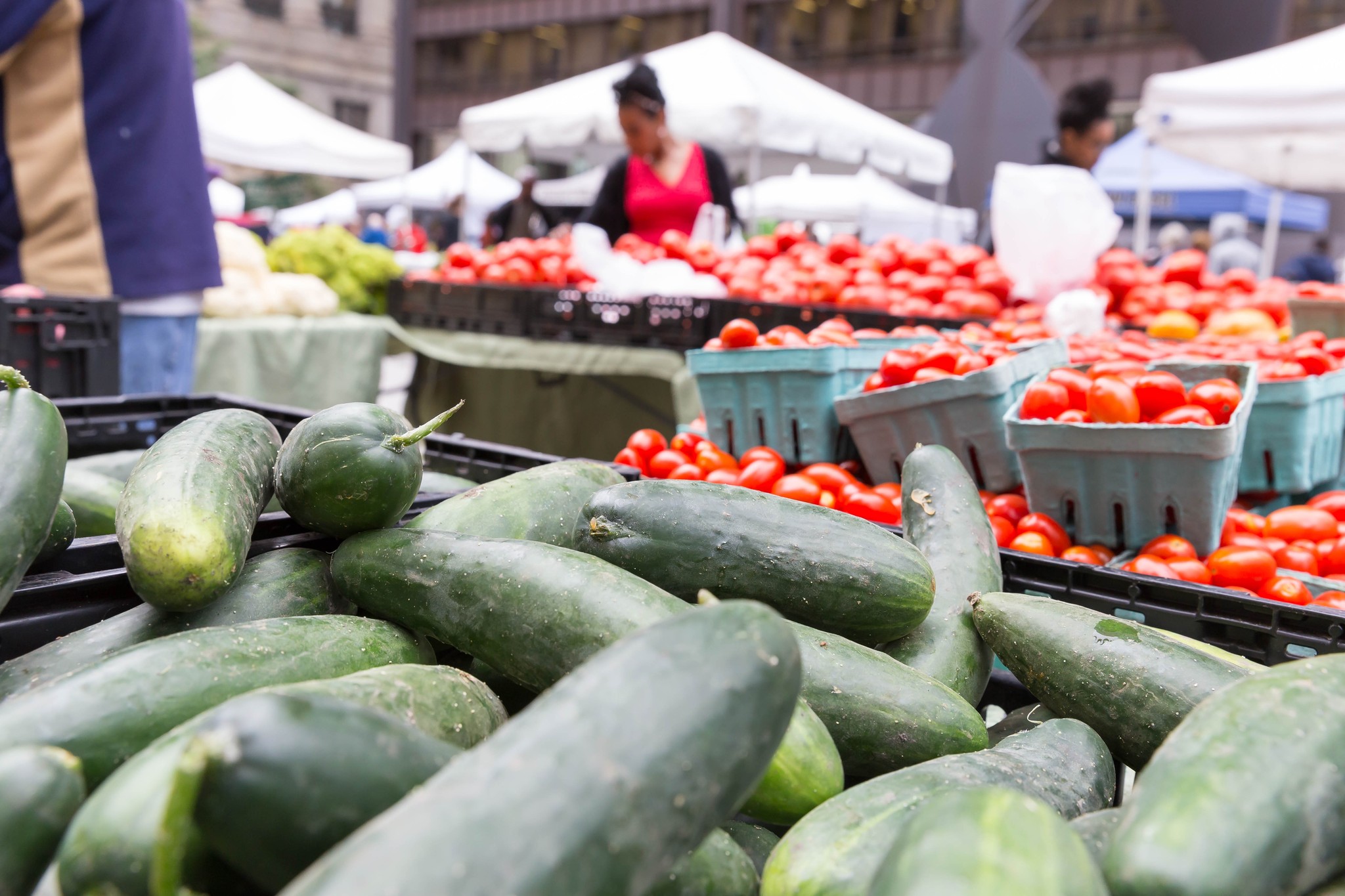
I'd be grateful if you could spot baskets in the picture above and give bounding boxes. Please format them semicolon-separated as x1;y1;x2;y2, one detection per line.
833;340;1070;492
685;344;945;471
386;279;974;351
1240;373;1345;497
1004;361;1258;555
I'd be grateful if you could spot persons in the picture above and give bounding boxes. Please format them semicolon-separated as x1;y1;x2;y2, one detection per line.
586;64;741;246
1;0;225;443
483;174;555;246
433;192;468;253
1042;79;1337;284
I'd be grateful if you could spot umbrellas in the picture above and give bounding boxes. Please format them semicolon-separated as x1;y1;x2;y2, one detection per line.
1091;126;1328;233
1130;24;1344;284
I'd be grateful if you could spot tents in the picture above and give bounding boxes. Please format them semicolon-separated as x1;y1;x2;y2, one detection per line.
194;31;977;295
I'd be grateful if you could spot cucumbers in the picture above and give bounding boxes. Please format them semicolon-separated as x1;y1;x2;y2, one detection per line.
0;367;1345;896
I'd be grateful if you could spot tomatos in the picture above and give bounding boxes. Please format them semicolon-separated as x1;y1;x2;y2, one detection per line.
402;221;1345;608
